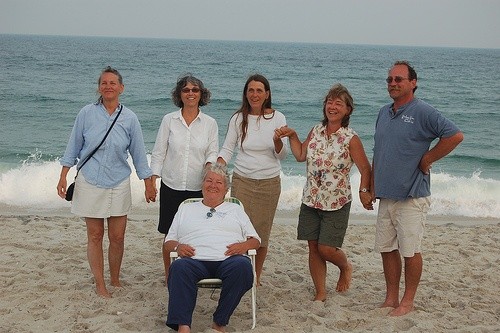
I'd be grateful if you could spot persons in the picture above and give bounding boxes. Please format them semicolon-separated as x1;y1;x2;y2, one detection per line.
281;82;372;302
218;73;288;285
368;61;464;317
162;161;261;332
56;65;157;298
149;75;220;281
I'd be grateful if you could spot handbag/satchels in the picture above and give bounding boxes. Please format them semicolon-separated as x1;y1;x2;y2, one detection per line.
65;182;74;201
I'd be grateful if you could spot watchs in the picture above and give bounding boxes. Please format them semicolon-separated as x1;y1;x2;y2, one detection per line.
173;244;180;251
358;187;370;192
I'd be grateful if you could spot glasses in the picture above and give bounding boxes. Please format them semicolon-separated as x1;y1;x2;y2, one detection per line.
206;208;216;218
182;86;200;93
386;77;409;84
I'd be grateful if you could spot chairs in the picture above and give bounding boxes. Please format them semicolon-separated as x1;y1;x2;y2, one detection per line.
169;197;258;330
